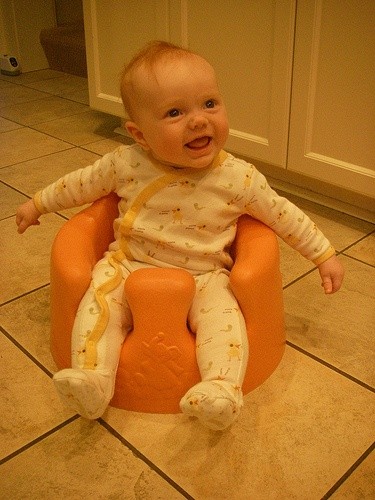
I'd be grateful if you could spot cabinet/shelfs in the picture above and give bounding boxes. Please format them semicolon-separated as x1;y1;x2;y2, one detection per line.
80;0;375;224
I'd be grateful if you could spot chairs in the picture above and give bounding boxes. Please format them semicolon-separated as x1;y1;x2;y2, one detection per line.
50;193;287;414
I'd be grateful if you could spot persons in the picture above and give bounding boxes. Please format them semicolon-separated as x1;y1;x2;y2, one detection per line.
15;43;346;433
